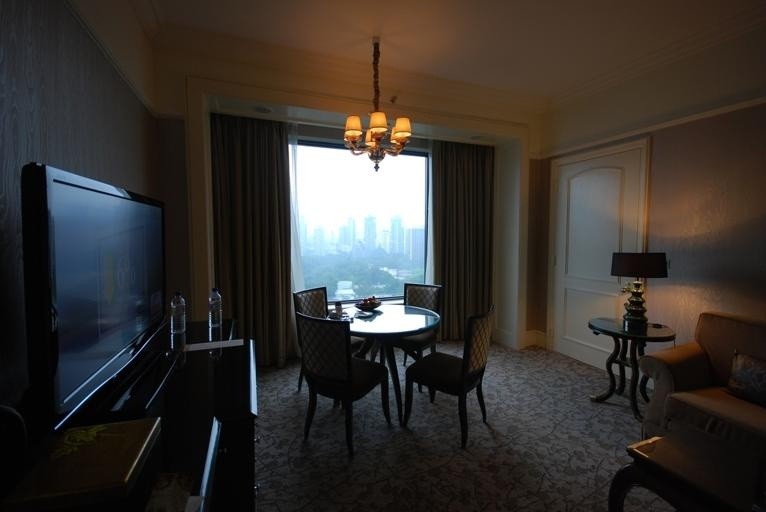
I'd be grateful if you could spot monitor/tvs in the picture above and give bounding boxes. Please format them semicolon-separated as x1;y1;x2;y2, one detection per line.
20;162;179;430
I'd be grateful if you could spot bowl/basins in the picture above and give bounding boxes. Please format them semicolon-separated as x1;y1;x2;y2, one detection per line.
355;302;381;310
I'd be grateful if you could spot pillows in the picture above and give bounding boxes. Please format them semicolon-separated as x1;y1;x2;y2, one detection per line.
722;347;765;408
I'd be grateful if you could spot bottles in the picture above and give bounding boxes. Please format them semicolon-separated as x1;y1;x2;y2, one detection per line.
208;287;224;326
172;335;187;362
209;328;223;361
134;292;147;331
170;289;188;333
335;301;343;317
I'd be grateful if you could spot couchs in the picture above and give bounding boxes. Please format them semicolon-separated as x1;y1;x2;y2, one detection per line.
640;310;765;455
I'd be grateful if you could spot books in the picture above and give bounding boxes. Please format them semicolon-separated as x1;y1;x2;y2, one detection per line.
1;414;164;506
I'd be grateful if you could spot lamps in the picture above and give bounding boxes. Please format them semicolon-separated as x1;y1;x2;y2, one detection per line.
610;250;669;332
342;34;414;171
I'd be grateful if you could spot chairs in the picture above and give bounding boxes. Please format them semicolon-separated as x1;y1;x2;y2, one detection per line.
395;282;443;393
291;287;364;406
400;304;494;449
293;311;391;457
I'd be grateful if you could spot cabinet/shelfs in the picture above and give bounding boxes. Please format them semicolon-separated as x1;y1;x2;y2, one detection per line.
2;318;265;512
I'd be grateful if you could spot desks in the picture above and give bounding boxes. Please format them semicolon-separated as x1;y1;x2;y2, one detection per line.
588;317;675;422
323;301;441;425
